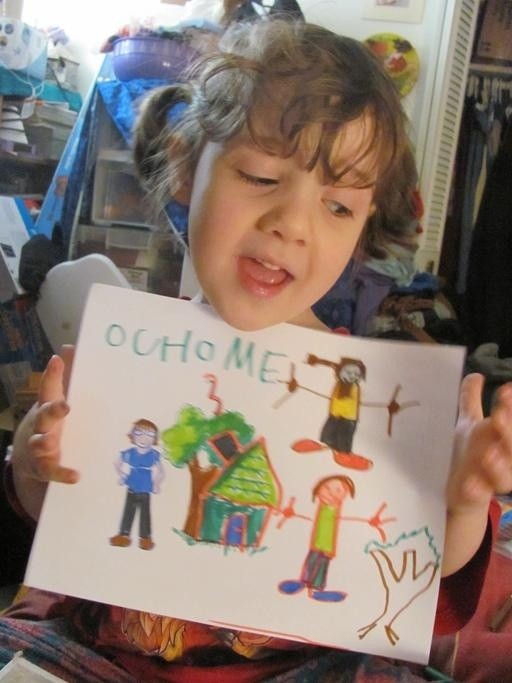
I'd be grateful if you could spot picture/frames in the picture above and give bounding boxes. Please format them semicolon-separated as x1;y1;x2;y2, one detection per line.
361;0;425;23
469;2;511;68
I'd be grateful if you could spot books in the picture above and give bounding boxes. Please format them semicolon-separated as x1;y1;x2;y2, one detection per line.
21;101;77;162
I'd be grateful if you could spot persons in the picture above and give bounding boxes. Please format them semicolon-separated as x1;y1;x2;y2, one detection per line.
0;20;512;683
280;476;354;602
110;419;167;549
292;355;369;470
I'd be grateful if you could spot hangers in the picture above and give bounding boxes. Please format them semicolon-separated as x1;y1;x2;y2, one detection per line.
460;76;512;120
